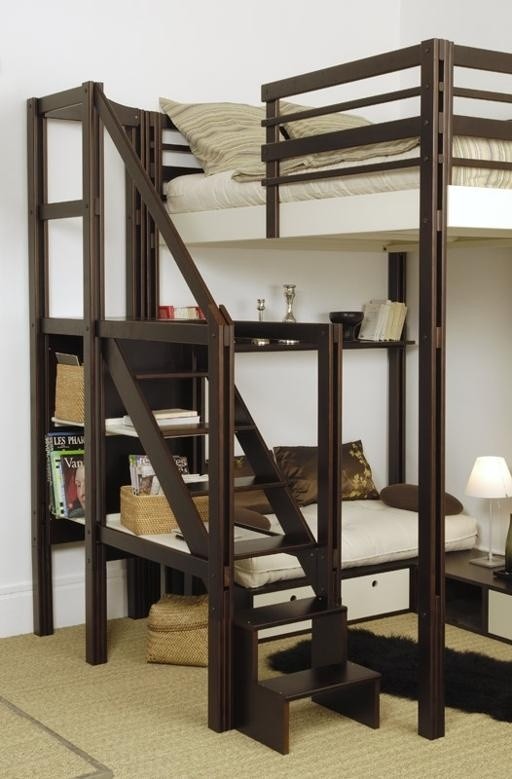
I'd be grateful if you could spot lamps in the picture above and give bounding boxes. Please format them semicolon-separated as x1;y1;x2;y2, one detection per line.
462;457;511;571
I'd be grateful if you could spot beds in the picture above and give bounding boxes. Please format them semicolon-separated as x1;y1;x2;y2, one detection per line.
84;501;475;731
159;112;512;252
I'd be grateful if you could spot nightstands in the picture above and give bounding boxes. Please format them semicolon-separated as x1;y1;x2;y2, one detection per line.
411;547;512;644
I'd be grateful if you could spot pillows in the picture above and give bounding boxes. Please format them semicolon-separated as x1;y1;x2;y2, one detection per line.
205;443;464;519
158;98;381;173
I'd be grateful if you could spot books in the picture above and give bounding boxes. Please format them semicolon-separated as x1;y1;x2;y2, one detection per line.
169;522;243;540
129;453;210;496
43;426;86;520
156;306;203;320
122;413;201;427
150;407;198;419
356;297;407;342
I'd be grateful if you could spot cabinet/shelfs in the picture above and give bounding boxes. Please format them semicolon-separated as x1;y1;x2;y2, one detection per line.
27;317;415;734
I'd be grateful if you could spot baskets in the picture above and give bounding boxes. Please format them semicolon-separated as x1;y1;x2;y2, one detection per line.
54;363;86;424
120;484;210;536
145;592;209;667
